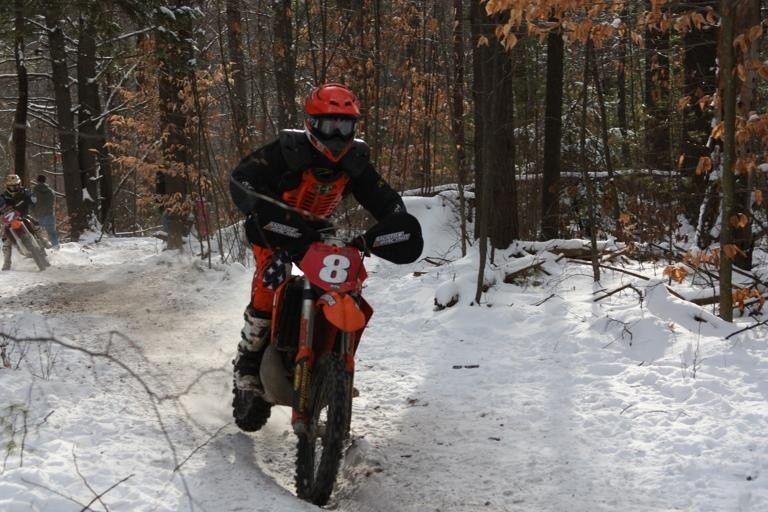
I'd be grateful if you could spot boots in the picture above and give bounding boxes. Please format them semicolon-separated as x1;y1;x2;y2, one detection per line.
2;246;11;270
235;307;271;392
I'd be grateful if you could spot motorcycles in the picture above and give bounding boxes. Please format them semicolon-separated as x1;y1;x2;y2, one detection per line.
0;190;50;270
226;185;425;507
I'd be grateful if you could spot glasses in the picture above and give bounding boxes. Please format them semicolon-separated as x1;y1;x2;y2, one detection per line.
304;114;359;134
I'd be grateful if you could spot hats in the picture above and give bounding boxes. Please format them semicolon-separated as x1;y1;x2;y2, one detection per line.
37;175;47;182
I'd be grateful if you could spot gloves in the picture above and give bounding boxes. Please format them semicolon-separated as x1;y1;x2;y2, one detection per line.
31;193;37;203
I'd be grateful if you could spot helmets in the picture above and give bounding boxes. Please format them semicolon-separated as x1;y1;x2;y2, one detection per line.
5;174;21;197
303;83;361;163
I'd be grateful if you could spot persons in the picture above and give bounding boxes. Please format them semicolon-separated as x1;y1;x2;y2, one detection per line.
230;83;407;392
31;175;59;251
0;174;53;270
